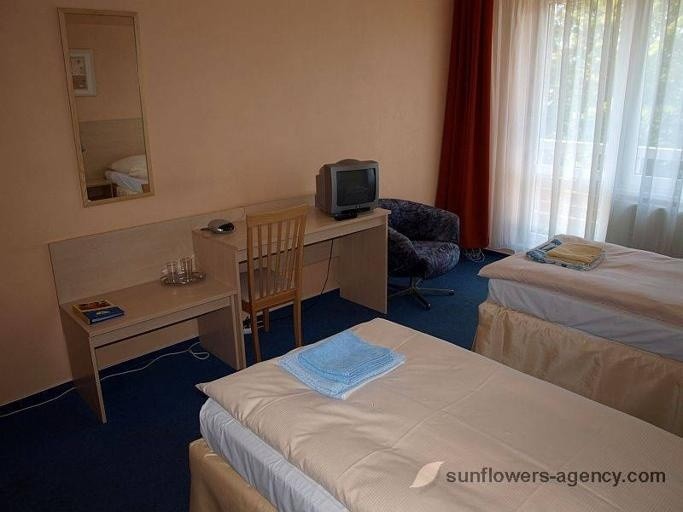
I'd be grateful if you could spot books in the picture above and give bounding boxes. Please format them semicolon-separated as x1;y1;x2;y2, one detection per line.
74;300;125;325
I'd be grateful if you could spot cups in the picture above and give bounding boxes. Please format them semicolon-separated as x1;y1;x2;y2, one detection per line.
165;261;179;286
180;256;195;283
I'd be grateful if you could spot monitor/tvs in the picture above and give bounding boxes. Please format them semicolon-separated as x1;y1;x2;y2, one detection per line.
316;159;379;215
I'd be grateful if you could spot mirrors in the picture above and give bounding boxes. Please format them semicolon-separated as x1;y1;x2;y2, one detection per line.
56;7;156;209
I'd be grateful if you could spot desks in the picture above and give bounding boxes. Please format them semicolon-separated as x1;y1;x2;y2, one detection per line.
49;194;393;424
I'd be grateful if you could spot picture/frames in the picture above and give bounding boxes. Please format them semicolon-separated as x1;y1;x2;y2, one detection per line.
67;49;95;96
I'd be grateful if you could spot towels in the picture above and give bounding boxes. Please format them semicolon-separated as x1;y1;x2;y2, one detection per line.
280;331;405;400
527;238;608;272
297;331;393;383
544;241;602;266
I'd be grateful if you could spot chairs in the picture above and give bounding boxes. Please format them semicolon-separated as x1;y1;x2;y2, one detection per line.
374;198;461;311
240;202;309;363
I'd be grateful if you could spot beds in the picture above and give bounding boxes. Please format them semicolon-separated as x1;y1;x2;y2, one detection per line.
472;235;683;437
104;153;152;195
189;318;683;512
